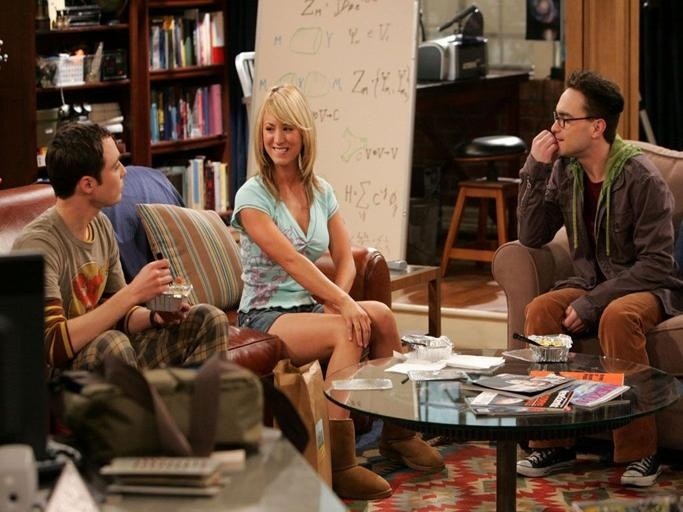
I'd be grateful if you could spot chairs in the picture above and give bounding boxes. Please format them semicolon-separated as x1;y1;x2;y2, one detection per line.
491;140;683;449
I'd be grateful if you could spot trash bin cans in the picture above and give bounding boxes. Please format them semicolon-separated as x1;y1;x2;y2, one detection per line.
407;198;439;265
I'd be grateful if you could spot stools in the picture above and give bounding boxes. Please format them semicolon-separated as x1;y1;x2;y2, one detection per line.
439;133;528;278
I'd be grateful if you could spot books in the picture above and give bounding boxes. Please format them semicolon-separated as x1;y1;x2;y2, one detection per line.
97;454;230;498
410;353;630;416
148;7;232;212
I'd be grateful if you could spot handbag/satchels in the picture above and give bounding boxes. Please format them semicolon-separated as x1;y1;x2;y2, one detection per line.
46;347;266;463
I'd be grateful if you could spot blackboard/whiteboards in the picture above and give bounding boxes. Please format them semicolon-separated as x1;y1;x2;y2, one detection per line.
247;0;419;270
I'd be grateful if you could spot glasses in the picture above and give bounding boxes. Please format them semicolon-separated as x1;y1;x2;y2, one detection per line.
549;102;604;129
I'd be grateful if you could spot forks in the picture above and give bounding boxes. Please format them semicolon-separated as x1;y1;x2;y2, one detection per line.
156;252;173;288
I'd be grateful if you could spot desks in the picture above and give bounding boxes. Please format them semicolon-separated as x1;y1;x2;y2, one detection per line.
102;428;348;511
412;67;530;241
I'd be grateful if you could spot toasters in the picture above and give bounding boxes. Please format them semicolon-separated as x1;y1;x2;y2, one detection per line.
415;32;488;81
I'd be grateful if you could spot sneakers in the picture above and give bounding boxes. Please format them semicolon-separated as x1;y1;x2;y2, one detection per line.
618;448;665;491
512;440;579;480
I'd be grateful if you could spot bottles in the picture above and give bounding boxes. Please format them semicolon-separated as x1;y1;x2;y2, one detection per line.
54;8;68;32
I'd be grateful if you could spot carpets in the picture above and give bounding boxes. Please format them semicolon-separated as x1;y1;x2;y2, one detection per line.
341;441;682;512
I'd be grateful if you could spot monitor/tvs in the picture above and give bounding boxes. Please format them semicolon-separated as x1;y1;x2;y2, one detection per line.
0;255;45;461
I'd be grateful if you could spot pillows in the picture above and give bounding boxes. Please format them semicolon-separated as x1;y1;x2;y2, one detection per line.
136;204;243;311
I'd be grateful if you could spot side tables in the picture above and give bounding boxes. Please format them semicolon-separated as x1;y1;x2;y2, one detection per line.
390;258;442;338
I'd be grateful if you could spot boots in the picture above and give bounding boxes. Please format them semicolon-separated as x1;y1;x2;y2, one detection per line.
376;417;446;476
322;416;393;506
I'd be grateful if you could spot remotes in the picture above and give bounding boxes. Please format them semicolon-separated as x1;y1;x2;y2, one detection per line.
100;455;221;476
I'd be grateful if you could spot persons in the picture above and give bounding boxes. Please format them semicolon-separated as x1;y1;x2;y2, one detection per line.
9;120;228;376
229;83;445;501
515;66;682;487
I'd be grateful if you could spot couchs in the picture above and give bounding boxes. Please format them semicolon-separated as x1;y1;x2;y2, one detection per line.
0;166;391;428
